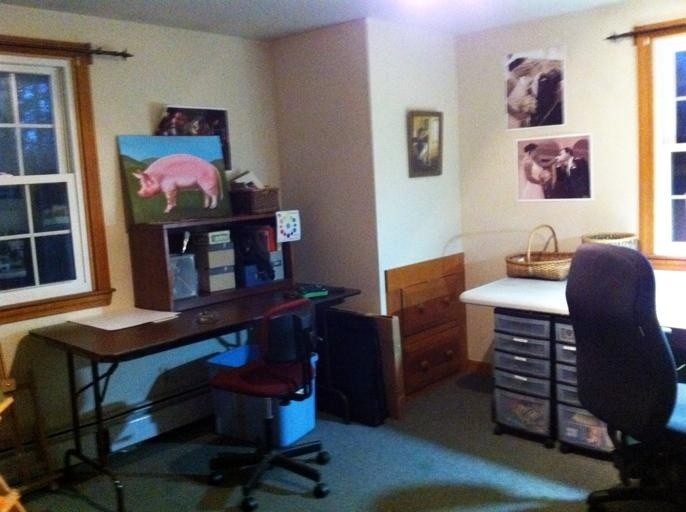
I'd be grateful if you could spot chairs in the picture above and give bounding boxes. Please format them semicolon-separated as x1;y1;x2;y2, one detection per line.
208;298;332;512
565;242;686;511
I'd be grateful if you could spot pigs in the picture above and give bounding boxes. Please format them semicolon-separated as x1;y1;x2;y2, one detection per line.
132;153;224;214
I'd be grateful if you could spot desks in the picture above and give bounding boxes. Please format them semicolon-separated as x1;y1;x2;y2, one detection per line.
29;283;361;511
459;259;686;463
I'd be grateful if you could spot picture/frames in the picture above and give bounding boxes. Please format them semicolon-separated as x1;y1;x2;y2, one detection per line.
500;43;568;133
158;105;232;171
514;132;595;203
406;108;445;179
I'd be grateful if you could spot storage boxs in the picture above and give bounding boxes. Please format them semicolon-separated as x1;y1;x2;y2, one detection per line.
201;344;320;449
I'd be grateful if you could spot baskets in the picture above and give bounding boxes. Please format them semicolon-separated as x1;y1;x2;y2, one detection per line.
505;225;575;280
581;233;638;250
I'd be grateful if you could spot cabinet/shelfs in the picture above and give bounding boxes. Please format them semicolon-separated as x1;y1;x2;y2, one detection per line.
384;253;468;396
127;213;296;313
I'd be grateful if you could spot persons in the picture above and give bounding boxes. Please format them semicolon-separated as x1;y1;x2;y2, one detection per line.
523;143;590;199
507;57;534;129
412;127;429;172
